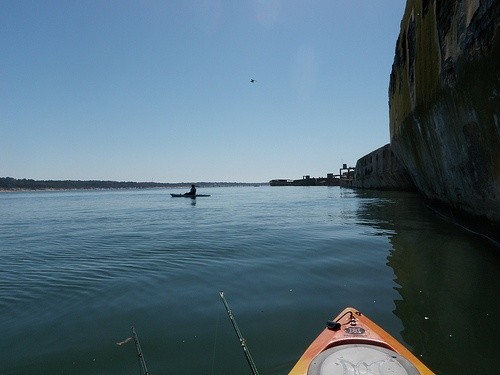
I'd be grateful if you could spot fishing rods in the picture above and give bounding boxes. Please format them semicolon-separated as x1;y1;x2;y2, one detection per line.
218;289;260;375
130;325;148;375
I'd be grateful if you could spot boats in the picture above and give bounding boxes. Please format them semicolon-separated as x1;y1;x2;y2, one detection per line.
169;192;212;199
287;306;436;375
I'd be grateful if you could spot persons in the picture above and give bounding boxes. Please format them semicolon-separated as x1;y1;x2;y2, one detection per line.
184;184;196;195
191;199;196;206
